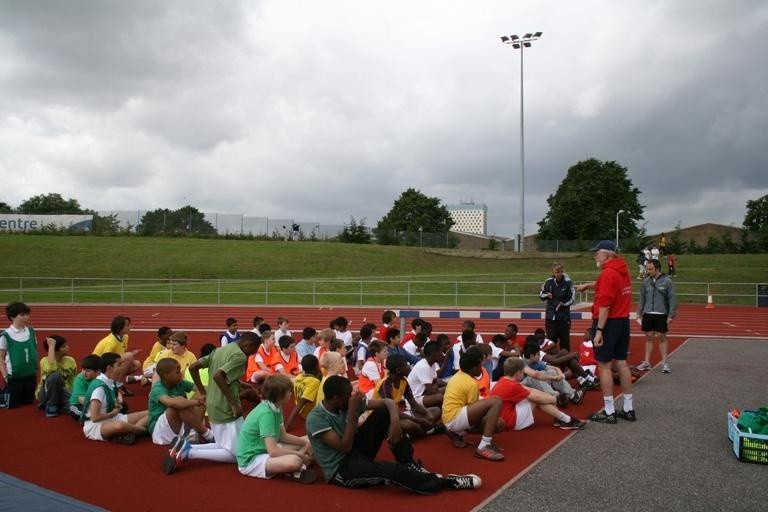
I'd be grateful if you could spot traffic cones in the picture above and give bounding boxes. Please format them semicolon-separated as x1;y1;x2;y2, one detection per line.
705;288;716;308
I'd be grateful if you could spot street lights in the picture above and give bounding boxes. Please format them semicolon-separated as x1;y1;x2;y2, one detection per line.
500;29;541;250
615;208;626;255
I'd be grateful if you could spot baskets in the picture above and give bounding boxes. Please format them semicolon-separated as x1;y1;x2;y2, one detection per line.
728;411;768;464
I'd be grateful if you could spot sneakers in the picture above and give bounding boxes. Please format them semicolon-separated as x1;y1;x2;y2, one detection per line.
404;422;505;488
39;374;199;473
279;469;317;483
552;361;671;429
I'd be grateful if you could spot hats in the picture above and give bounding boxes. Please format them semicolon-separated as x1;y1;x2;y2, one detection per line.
589;239;619;254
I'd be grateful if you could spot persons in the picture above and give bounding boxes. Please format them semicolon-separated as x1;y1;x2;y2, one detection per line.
539;263;576;353
658;232;667;256
282;222;300;241
667;251;677;278
573;240;636;425
637;244;667;280
636;259;677;373
0;302;600;495
